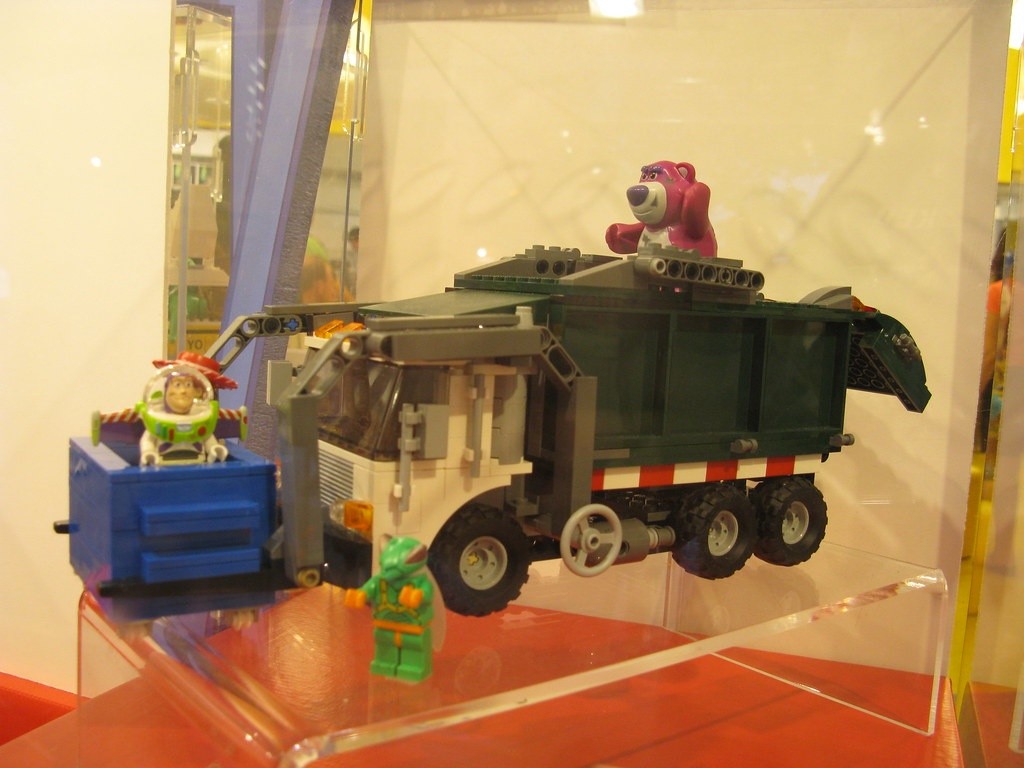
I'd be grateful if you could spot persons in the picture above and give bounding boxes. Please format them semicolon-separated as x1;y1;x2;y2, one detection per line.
297;226;360;302
978;225;1016;444
214;131;233;282
137;362;229;469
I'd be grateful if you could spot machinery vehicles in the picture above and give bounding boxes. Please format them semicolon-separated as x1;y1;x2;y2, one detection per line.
54;243;932;618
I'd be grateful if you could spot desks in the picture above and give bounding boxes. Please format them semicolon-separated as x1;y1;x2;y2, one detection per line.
0;586;965;768
